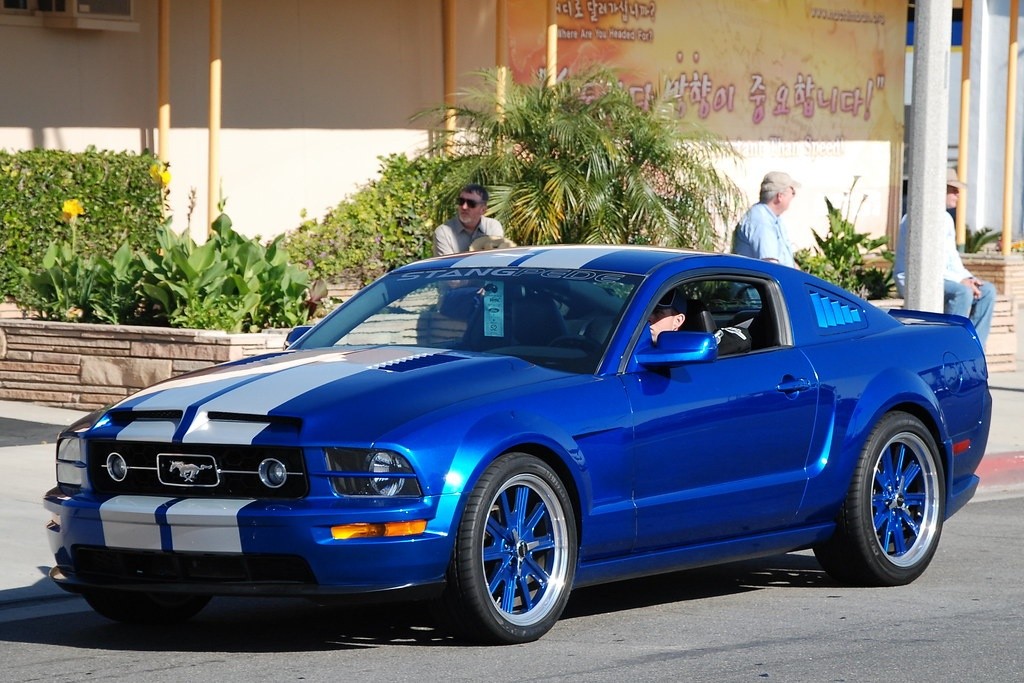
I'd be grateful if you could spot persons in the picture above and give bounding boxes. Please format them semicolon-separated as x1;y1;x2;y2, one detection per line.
731;171;804;271
649;287;752;356
432;186;519;353
894;167;997;352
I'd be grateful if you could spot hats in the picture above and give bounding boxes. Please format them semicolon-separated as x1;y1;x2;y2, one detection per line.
760;172;800;192
657;287;687;316
946;168;967;189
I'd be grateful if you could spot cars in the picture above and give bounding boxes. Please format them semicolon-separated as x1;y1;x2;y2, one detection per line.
40;245;993;645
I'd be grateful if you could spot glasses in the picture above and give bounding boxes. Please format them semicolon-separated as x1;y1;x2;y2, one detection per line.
456;197;486;209
